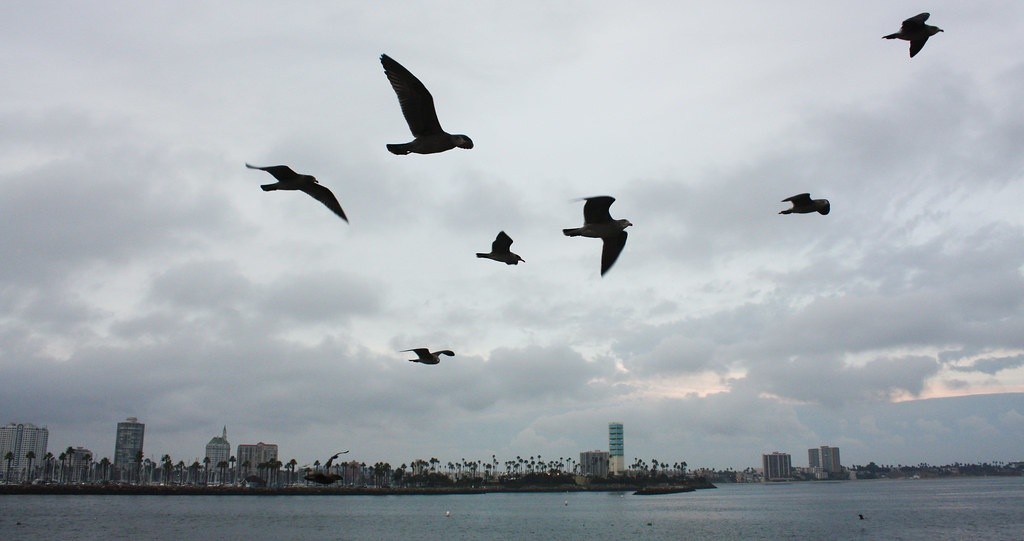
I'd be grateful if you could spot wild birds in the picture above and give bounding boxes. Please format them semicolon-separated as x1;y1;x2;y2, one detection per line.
246;163;350;223
380;53;474;155
859;514;867;520
476;230;526;265
563;195;633;276
881;12;945;57
778;192;831;216
400;348;455;364
